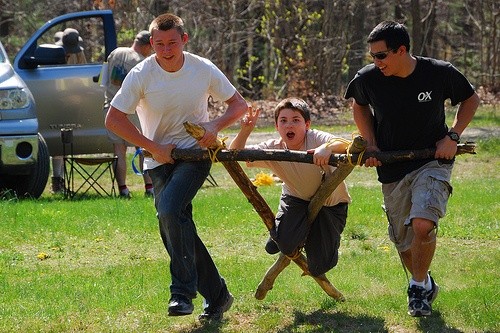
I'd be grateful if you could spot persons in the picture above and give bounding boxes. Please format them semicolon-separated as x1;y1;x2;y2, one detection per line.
50;28;88;196
229;97;352;277
103;31;154;197
344;20;479;316
103;13;249;328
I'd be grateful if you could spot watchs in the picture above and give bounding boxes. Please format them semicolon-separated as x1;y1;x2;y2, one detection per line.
446;132;460;142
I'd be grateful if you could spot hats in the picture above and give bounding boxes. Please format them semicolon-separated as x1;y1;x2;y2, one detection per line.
54;27;84;53
140;31;151;41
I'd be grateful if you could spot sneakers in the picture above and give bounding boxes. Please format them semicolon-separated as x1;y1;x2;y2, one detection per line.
426;270;440;307
406;285;432;316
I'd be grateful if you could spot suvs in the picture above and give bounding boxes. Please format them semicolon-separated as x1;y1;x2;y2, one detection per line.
0;9;118;201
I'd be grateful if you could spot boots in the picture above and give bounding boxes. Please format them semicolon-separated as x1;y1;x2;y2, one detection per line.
51;177;74;196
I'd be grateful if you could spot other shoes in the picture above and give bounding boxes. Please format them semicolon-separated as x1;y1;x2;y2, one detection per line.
265;237;280;254
118;185;134;201
199;289;234;323
145;184;153;197
168;294;194;317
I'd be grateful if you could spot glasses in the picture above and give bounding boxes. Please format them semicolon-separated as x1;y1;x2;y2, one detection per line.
370;48;397;60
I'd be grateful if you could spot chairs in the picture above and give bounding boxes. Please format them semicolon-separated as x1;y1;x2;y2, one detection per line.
60;128;118;200
